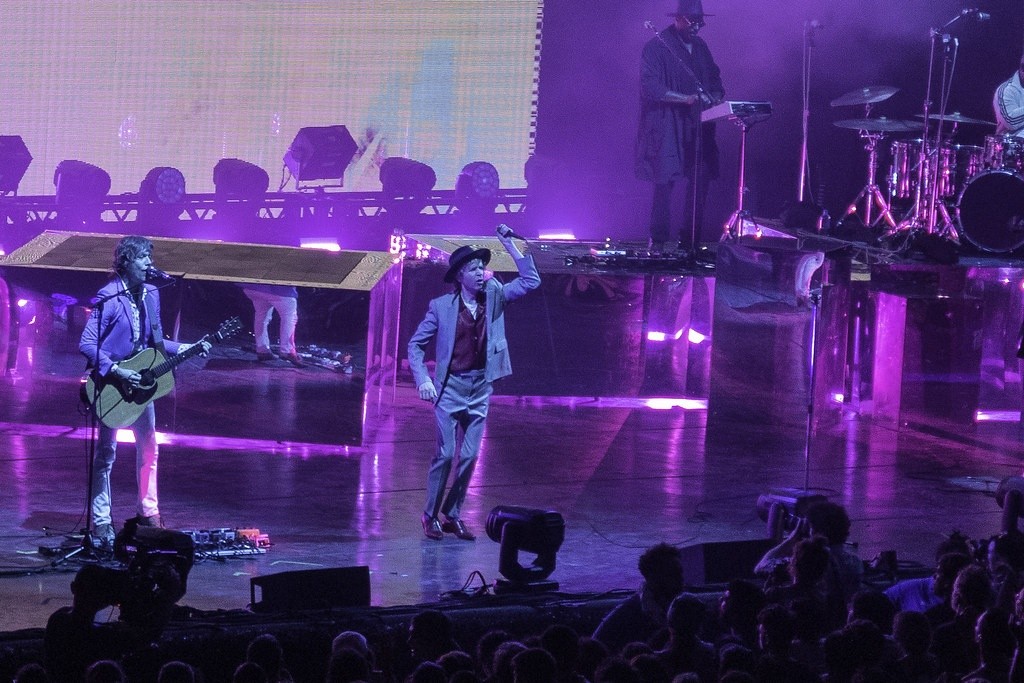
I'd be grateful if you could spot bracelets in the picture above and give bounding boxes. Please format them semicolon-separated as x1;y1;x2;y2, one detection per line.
110;364;118;374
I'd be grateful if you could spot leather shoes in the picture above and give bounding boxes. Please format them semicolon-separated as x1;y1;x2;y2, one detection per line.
421;512;445;542
442;516;476;540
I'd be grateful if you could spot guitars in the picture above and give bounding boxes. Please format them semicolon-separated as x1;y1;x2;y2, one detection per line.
79;315;248;430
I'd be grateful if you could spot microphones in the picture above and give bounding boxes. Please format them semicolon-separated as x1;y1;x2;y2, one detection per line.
497;226;527;240
644;21;655;29
809;19;824;30
971;12;990;20
146;265;173;281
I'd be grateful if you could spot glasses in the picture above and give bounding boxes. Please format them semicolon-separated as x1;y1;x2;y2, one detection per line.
683;15;706;28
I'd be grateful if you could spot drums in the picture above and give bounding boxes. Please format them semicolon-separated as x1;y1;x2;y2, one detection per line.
954;144;985;183
983;134;1024;171
889;140;956;200
955;167;1024;255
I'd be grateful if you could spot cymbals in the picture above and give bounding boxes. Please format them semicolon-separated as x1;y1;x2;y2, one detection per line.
830;84;903;107
832;118;932;132
913;114;999;127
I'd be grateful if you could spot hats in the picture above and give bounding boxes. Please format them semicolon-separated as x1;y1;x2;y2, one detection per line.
444;244;492;285
667;1;715;18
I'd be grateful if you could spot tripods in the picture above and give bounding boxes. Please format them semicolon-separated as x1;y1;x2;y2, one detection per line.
51;277;158;568
720;119;762;243
834;8;978;247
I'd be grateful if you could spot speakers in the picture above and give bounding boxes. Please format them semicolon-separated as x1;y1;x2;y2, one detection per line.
681;537;777;590
283;126;357;181
250;566;371;614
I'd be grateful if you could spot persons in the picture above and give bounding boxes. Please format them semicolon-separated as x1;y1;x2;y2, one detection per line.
78;236;213;550
408;224;542;542
639;0;727;264
993;54;1024;141
1;494;1024;682
244;282;309;370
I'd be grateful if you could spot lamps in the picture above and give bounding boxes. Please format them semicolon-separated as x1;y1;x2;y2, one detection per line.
380;157;436;202
53;160;112;204
213;158;269;202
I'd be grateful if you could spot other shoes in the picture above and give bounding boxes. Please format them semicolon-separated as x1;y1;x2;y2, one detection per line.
142;516;166;529
94;524;115;539
